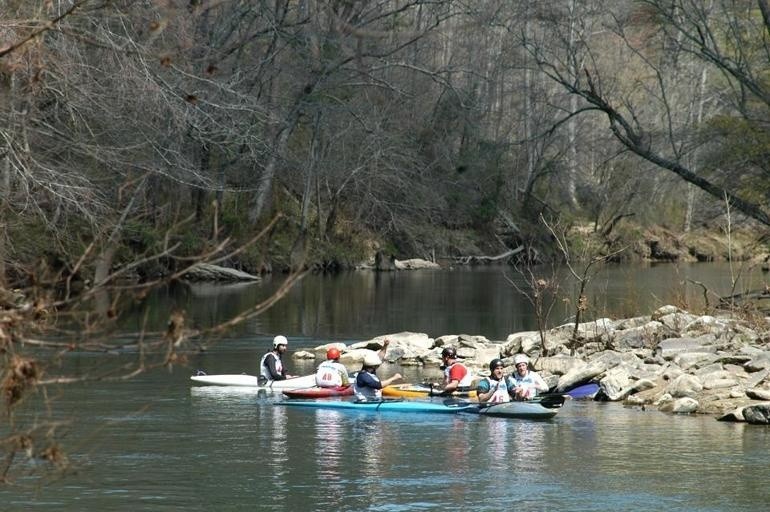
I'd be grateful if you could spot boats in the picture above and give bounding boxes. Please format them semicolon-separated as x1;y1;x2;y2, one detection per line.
281;383;357;399
269;401;477;416
186;368;359;388
476;404;562;420
379;384;477;400
533;391;566;410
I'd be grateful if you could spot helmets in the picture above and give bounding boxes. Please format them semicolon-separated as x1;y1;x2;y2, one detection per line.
362;353;383;366
490;358;505;371
515;354;529;367
441;347;458;359
272;335;288;349
326;347;341;359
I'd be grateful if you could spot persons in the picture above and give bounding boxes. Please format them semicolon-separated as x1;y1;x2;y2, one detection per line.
512;355;549;399
260;335;299;380
353;337;402;400
315;348;349;388
477;359;523;404
431;347;472;396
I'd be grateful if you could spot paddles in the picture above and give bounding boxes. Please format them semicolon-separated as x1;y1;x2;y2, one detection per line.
533;384;598;395
445;398;507;407
